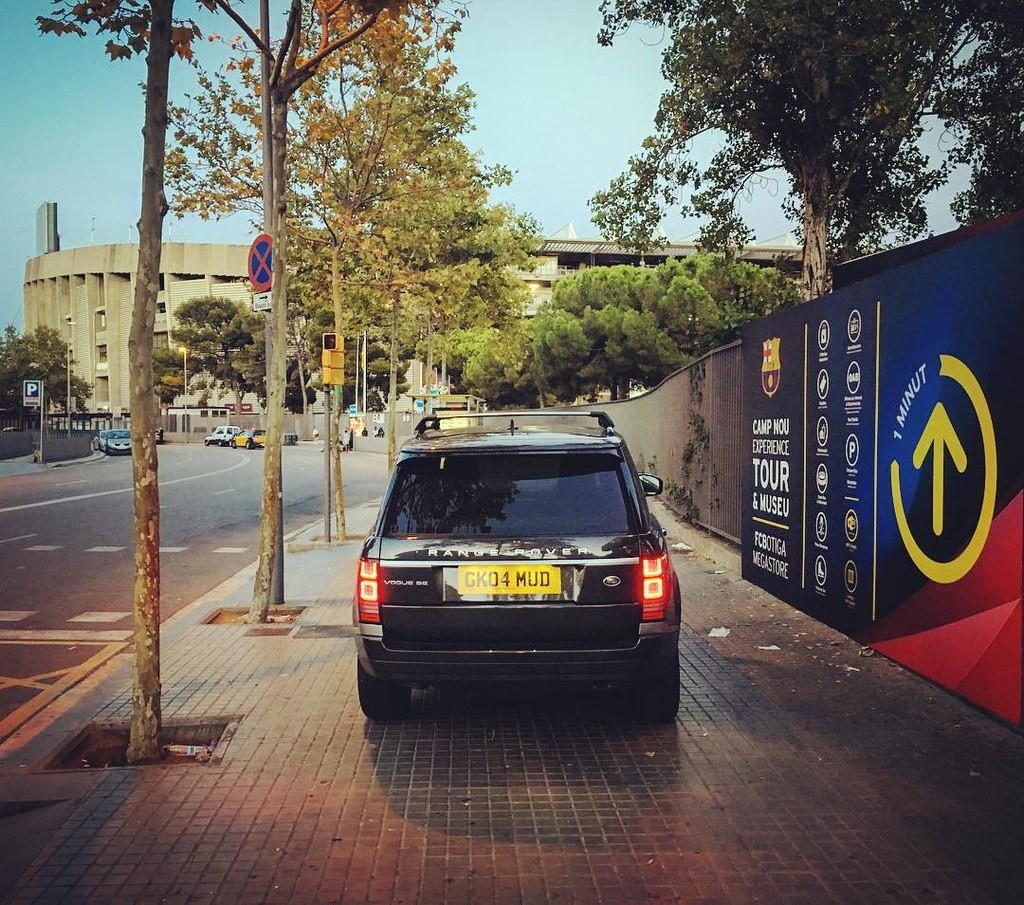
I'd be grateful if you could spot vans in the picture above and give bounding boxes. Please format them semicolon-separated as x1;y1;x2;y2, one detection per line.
206;426;240;447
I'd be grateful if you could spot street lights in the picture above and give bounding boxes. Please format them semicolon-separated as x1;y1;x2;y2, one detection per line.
180;345;191;445
67;322;80;438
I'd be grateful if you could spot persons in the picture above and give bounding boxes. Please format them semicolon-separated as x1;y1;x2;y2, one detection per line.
157;427;165;443
362;426;386;438
312;425;320;442
348;427;357;450
343;429;349;452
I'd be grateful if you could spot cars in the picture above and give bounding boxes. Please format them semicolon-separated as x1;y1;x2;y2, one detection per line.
104;429;132;455
93;430;114;452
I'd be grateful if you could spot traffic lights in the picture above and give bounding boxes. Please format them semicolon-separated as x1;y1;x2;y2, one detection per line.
322;332;344;384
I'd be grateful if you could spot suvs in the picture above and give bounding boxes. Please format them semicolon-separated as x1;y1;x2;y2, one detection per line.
353;411;682;725
233;429;266;449
